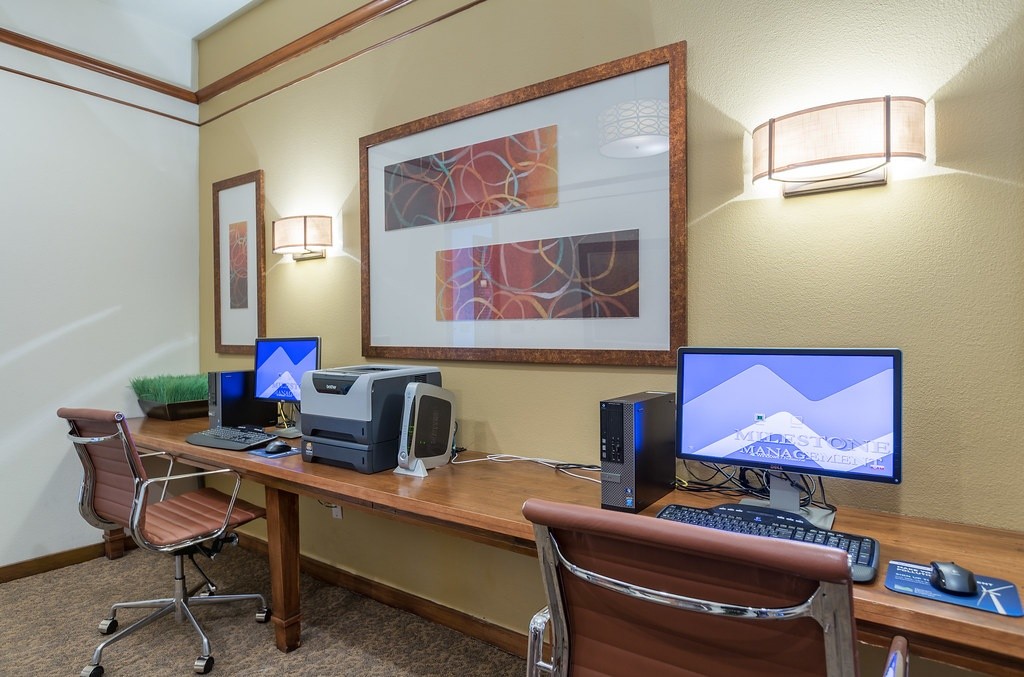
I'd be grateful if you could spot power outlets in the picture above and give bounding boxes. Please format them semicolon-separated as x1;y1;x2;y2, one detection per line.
330;504;343;519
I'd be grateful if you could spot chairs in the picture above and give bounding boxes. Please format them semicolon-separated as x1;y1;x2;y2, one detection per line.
522;497;911;677
57;407;272;677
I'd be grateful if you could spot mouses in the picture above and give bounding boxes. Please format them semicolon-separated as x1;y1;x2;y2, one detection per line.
929;561;978;596
265;440;291;453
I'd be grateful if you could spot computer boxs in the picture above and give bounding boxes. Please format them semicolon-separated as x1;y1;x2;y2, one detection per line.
208;370;278;430
600;391;677;514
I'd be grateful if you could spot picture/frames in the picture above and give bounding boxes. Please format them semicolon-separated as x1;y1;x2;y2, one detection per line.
359;41;689;368
211;169;267;356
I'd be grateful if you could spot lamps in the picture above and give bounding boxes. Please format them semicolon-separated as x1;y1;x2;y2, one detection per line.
272;215;332;262
752;95;926;198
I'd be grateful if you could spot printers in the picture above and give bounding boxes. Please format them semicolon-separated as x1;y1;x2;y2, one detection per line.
301;364;442;474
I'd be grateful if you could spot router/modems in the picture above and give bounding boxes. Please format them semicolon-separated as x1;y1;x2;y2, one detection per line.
397;381;456;470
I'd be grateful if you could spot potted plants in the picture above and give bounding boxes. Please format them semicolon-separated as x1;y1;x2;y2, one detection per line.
130;372;209;421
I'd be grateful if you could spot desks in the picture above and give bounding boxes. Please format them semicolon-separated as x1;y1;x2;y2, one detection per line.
125;416;1024;677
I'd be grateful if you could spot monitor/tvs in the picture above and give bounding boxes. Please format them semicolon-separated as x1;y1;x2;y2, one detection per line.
253;335;321;438
676;346;902;531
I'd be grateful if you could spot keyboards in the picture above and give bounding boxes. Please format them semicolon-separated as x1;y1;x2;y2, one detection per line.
186;426;278;451
656;503;881;582
